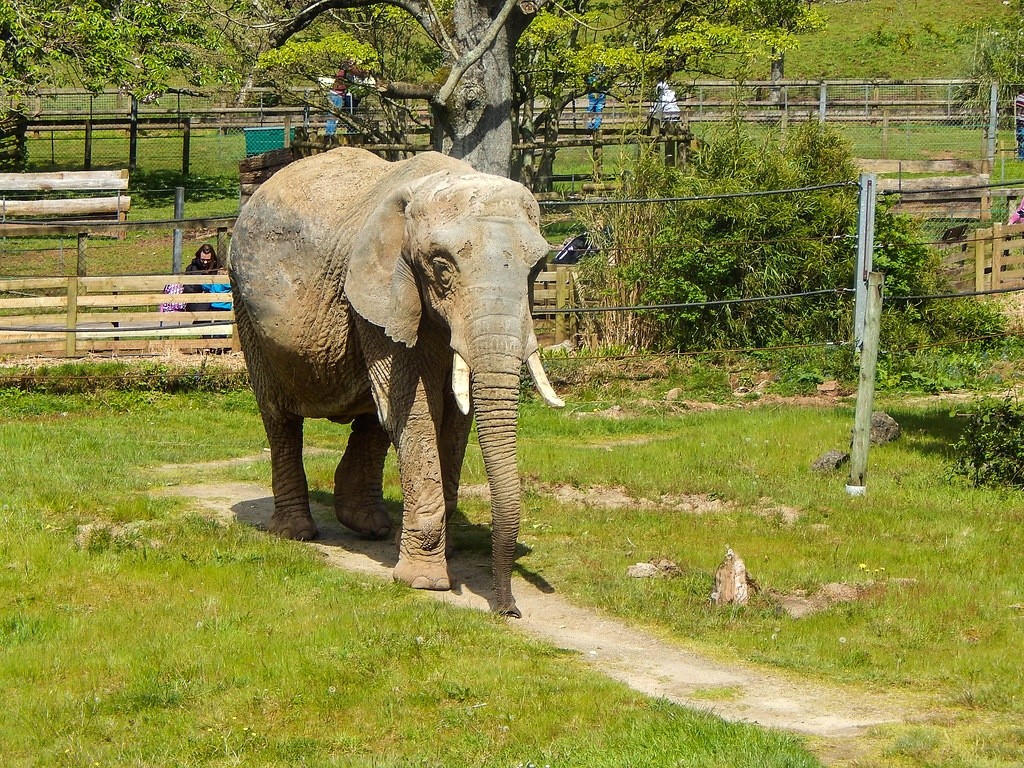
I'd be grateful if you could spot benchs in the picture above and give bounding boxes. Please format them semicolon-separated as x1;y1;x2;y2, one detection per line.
0;169;132;242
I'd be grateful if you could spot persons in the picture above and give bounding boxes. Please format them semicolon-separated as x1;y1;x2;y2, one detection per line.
649;81;680;120
163;284;186;312
1016;92;1024;160
202;267;232;338
183;243;218;324
326;57;368;134
585;64;607;128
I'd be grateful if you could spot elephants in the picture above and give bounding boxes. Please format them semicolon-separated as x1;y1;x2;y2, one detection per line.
226;146;566;620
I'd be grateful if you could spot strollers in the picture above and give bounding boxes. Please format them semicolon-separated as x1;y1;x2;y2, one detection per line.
542;232;596;287
